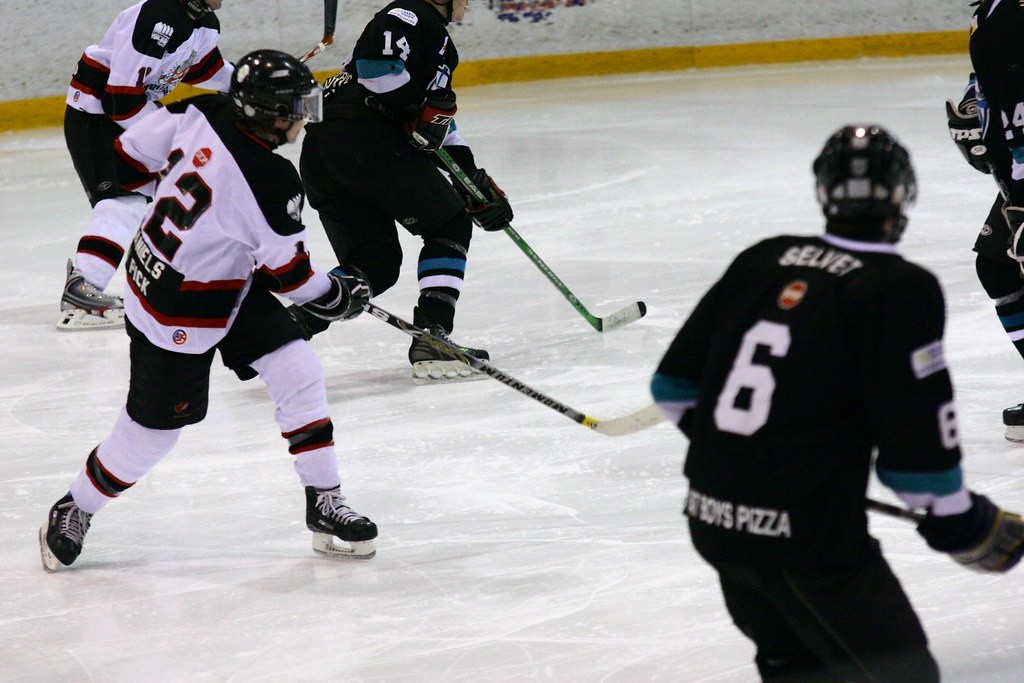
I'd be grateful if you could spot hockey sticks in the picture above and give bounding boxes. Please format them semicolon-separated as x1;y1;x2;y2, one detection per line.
865;496;928;523
297;0;337;64
359;299;669;437
434;146;647;333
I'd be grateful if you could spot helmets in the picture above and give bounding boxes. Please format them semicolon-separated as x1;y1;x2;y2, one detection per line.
230;50;316;129
813;126;914;239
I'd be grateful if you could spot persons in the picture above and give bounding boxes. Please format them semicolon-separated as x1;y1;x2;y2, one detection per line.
946;0;1024;441
291;1;514;387
55;0;238;330
650;125;1024;683
35;48;379;574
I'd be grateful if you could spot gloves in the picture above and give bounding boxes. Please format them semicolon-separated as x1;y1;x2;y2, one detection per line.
403;94;458;153
944;99;993;174
301;274;370;323
1002;202;1024;277
448;168;515;232
917;491;1024;574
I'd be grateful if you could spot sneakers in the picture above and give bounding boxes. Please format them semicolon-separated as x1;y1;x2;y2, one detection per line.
38;492;97;573
305;484;378;560
57;259;126;329
1003;403;1024;440
408;306;490;385
